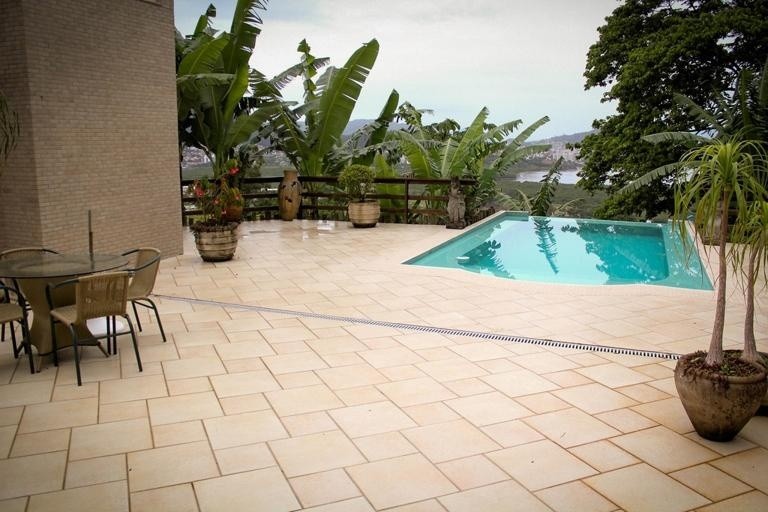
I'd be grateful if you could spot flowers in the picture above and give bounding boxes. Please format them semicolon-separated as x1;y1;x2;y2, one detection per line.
185;159;251;226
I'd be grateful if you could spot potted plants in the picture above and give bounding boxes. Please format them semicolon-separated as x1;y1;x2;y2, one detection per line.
672;132;768;442
723;201;768;416
337;164;381;228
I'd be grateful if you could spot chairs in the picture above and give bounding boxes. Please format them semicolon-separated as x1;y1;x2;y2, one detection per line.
0;247;61;355
0;280;34;374
113;246;167;355
45;271;143;386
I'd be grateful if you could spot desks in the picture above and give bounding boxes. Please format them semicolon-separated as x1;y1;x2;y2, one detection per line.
0;253;129;372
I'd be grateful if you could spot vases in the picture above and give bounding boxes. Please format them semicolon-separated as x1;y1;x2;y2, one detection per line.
192;221;239;262
278;170;302;221
